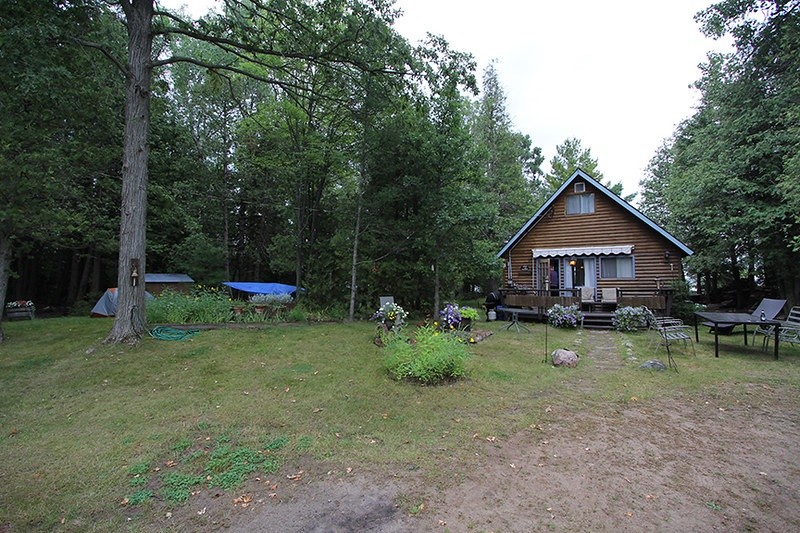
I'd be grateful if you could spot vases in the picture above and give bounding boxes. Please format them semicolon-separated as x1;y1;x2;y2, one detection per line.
458;317;472;332
231;304;291;317
6;307;36;320
385;317;396;329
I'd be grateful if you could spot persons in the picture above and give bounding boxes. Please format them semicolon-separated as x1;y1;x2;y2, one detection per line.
550;271;559;297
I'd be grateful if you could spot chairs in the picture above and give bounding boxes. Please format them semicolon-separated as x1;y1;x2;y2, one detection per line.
642;305;698;357
752;305;800;353
580;287;596;312
599;288;619;311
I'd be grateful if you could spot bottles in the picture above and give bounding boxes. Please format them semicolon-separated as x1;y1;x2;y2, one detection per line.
761;310;766;321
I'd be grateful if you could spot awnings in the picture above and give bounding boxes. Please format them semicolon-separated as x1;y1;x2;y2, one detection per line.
531;245;635;258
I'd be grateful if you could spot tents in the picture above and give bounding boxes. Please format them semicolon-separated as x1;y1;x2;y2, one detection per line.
91;287;155;318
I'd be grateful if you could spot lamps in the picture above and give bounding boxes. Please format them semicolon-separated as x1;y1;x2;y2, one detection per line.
570;256;578;266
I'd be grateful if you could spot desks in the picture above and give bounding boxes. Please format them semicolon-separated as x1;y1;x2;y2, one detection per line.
500;308;537;334
692;311;781;361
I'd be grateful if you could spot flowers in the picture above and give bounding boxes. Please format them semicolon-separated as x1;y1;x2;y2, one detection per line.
458;306;479;321
5;300;35;308
229;293;294;309
369;301;410;322
